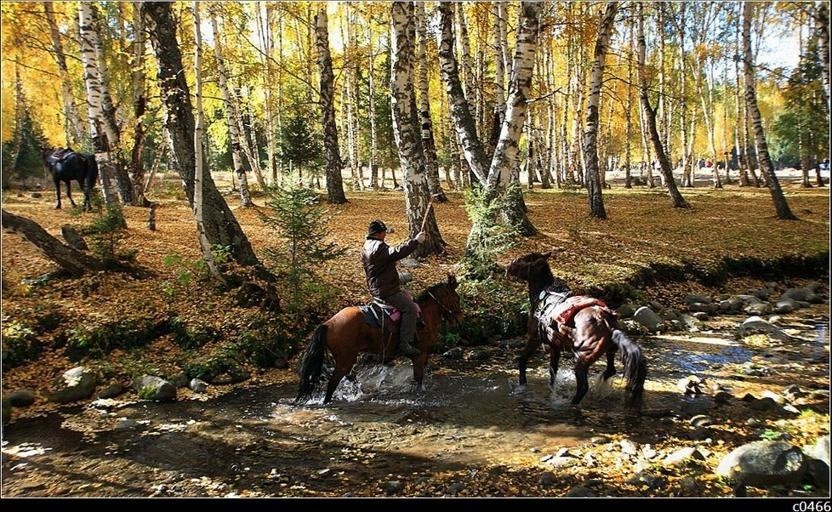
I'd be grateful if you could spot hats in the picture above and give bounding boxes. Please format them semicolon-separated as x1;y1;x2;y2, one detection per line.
367;220;387;236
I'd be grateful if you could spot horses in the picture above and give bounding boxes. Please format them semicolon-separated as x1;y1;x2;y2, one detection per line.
508;252;648;413
294;273;465;405
40;147;98;212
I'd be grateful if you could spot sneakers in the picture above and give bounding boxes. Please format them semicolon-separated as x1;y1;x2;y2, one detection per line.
400;347;422;358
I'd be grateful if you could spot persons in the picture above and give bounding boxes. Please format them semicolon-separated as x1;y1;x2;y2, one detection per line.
359;217;429;360
